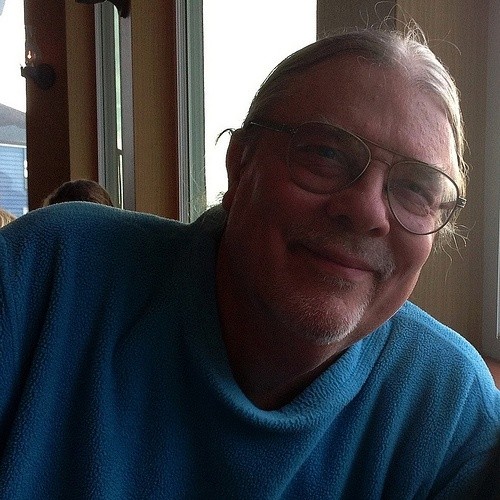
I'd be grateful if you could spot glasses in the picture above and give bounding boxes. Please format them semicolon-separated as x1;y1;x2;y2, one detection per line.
246;120;467;236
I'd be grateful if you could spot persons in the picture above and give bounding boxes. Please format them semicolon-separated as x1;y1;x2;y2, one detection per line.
0;30;499;499
0;209;19;231
42;178;115;209
